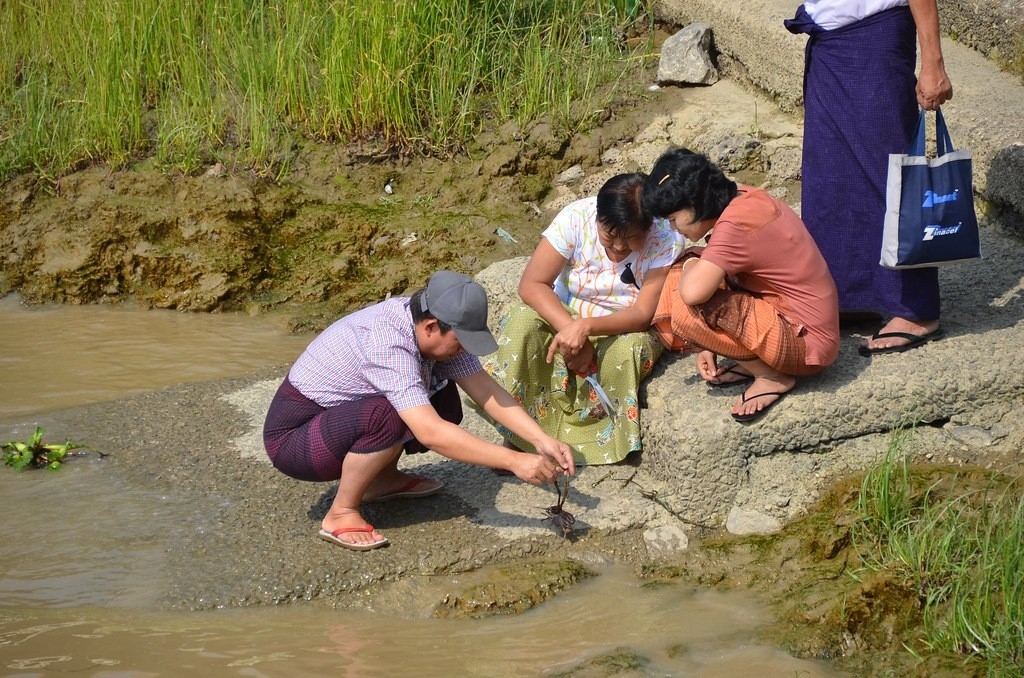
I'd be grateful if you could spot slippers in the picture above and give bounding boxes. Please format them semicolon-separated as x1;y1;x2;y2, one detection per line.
732;382;800;421
708;364;755;387
318;522;389;552
362;472;445;502
858;325;944;355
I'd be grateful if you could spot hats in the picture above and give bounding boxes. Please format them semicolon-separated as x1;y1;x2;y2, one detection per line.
420;270;500;358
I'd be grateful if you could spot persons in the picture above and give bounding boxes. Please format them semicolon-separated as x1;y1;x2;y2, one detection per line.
492;172;686;476
263;270;574;551
783;0;953;353
644;148;840;420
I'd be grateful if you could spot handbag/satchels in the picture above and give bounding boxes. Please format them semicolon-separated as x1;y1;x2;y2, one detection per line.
878;107;983;270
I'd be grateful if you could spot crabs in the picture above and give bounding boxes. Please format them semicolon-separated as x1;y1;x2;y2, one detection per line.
540;504;575;536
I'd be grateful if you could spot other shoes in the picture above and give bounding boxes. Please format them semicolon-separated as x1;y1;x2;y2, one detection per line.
495;466;513;476
